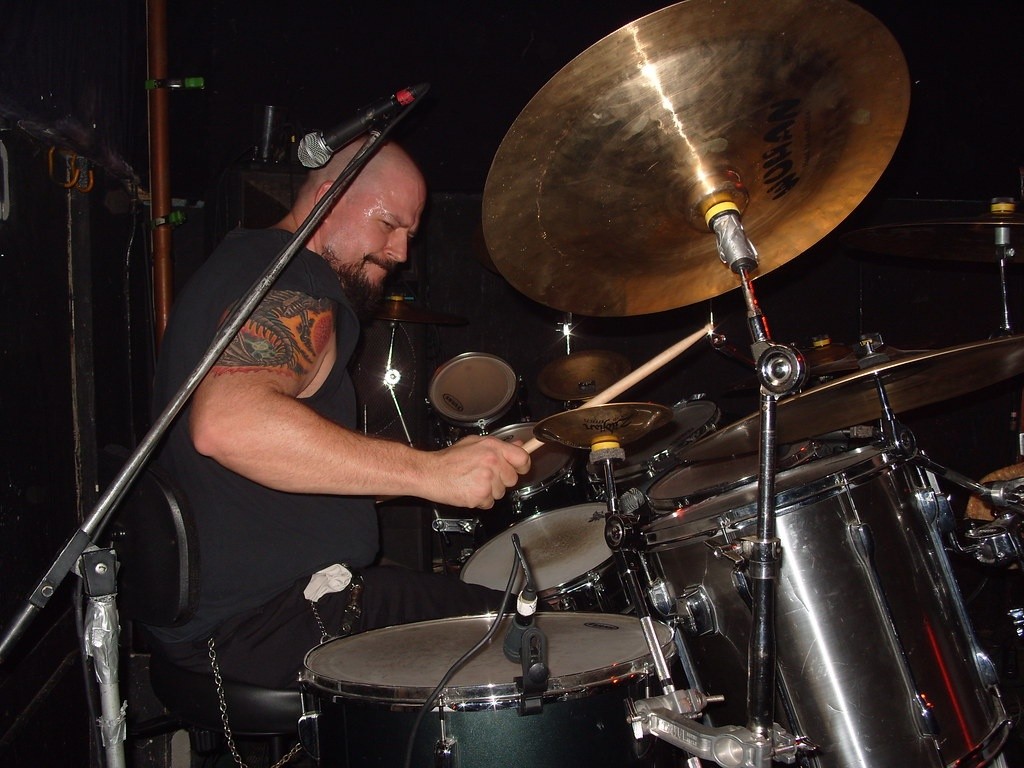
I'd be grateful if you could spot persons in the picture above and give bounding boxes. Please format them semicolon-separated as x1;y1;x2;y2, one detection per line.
117;136;531;692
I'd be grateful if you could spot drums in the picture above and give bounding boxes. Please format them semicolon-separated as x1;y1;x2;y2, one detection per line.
297;607;676;768
609;440;1011;767
472;421;585;540
451;501;652;614
647;435;822;517
425;351;526;447
585;399;723;498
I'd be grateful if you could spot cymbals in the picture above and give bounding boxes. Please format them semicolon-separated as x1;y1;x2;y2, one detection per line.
537;348;632;400
838;214;1024;265
481;1;912;319
364;301;469;324
717;341;936;392
533;403;673;449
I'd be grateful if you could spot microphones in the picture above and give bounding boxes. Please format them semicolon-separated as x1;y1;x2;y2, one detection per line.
504;580;539;663
296;83;428;168
